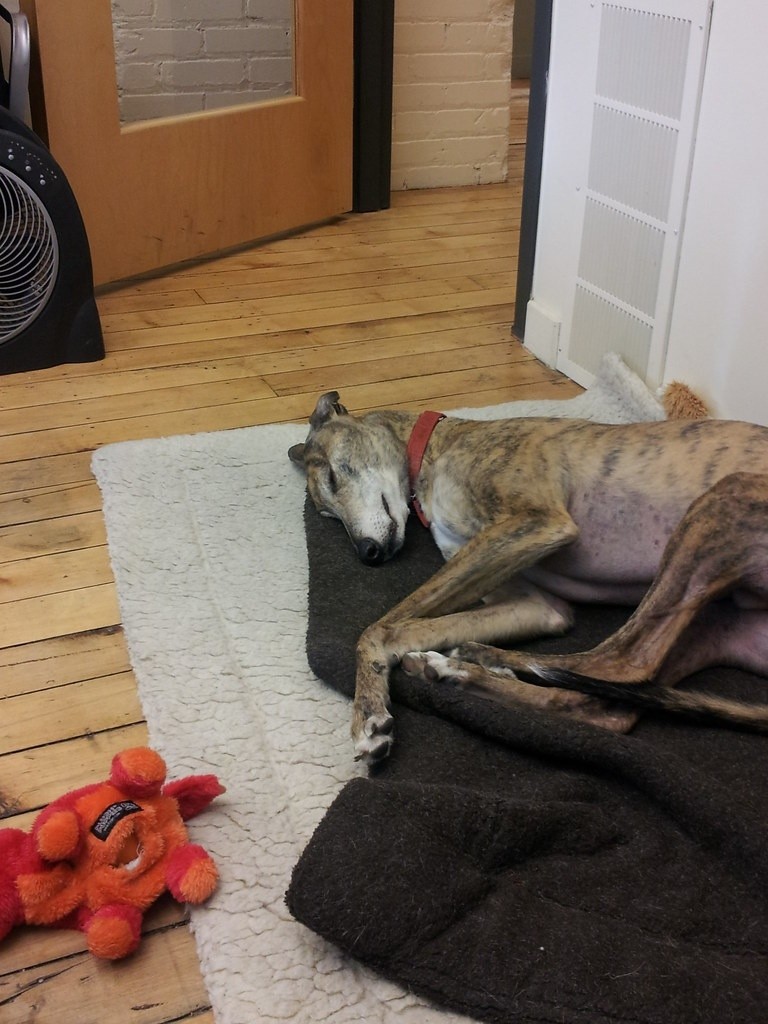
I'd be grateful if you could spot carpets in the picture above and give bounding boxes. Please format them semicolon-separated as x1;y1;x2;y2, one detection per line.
88;349;667;1024
282;465;768;1024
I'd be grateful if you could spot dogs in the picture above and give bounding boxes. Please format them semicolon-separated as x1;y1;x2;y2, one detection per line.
287;390;768;762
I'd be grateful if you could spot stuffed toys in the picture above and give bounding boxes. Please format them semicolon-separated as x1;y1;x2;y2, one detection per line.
0;747;227;961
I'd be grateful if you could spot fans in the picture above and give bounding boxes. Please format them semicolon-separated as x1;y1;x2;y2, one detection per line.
0;103;106;376
